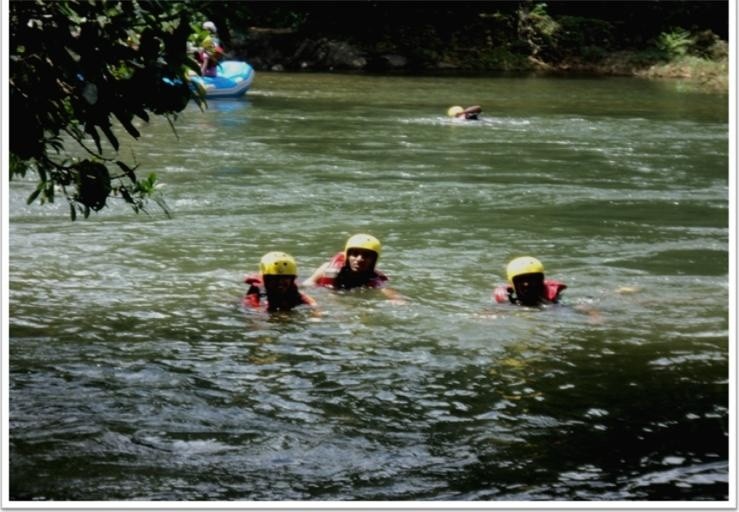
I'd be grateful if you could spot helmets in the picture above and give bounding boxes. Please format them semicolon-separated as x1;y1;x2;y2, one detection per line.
203;22;216;33
506;256;545;292
447;105;463;117
259;252;297;276
343;232;382;272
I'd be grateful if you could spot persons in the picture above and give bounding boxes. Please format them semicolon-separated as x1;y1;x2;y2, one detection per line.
302;233;410;308
240;250;323;324
186;20;222;74
200;36;226;77
490;256;607;326
446;104;484;122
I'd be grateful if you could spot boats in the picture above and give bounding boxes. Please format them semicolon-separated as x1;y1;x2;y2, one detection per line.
70;60;260;101
